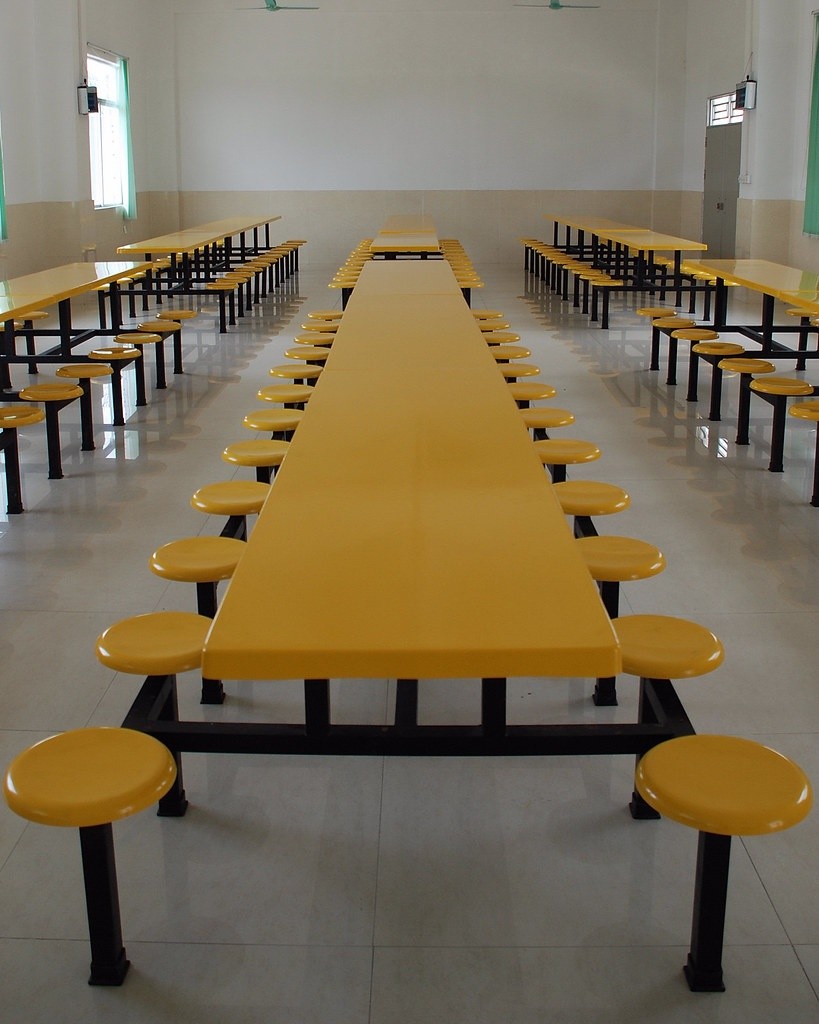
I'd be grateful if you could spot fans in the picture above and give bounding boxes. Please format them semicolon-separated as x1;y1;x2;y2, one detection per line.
513;0;600;9
237;0;320;12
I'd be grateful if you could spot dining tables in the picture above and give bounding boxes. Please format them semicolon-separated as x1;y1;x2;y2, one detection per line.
368;232;445;261
544;214;707;291
0;294;58;451
351;260;463;294
684;259;819;299
379;212;436;234
147;294;668;761
0;261;153;364
117;214;282;295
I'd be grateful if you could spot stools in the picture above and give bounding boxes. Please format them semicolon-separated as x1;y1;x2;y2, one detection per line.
671;329;720;403
299;320;342;333
202;272;307;382
437;238;484;309
488;345;531;363
258;385;315;409
15;311;49;375
471;309;505;320
95;609;213;820
89;347;142;426
190;480;272;542
636;307;678;371
308;310;344;321
573;534;666;705
54;364;115;452
606;612;724;822
810;318;819;351
476;320;511;332
784;308;818;371
718;358;776;446
0;322;25;389
778;290;819;316
517;237;624;330
205;240;307;334
599;237;742;326
293;333;336;347
749;377;815;473
1;370;202;623
633;732;812;995
0;406;46;515
220;439;290;484
19;382;85;480
533;437;601;485
148;537;247;705
652;318;696;385
632;382;819;605
498;362;540;384
692;343;745;422
327;238;375;311
114;332;162;407
137;321;183;389
483;331;521;347
5;724;176;990
519;406;576;441
91;238;226;329
269;363;323;385
156;310;195;375
508;382;557;410
284;347;331;365
242;407;305;442
788;400;819;508
516;269;622;378
552;480;629;539
